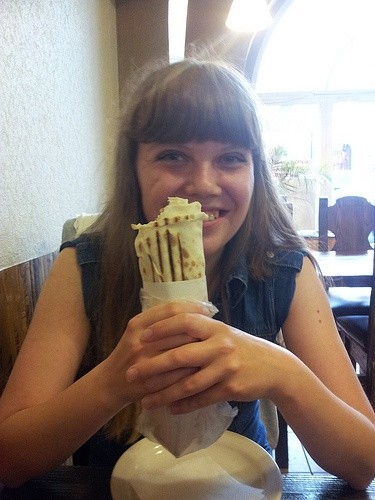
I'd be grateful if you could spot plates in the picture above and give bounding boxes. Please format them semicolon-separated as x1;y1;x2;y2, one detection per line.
110;432;282;500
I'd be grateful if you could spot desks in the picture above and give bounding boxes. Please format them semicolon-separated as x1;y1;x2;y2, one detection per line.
297;229;336;251
308;250;375;287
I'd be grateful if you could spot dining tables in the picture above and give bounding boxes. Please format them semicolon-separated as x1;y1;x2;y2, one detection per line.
0;463;375;500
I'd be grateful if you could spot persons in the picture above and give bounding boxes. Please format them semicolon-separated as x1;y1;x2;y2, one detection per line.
0;57;375;491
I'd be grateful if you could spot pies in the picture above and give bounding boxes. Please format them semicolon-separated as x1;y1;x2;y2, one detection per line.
131;196;208;283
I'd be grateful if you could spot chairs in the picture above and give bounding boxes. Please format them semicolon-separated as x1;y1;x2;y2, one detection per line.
0;248;83;396
318;196;375;315
333;312;375;409
